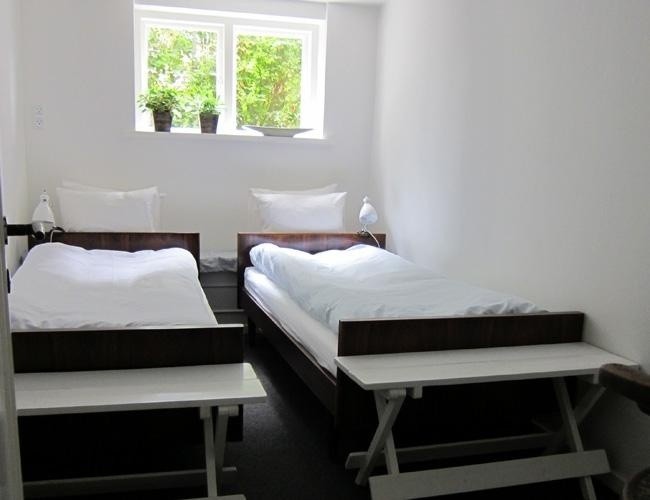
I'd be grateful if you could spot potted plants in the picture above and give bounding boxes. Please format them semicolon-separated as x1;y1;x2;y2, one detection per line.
200;98;219;133
138;81;180;132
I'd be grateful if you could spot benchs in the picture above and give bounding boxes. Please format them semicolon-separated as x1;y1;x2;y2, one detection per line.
333;342;640;500
13;363;267;500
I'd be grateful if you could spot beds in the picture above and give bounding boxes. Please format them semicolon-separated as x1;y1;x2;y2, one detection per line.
237;234;584;465
11;233;244;443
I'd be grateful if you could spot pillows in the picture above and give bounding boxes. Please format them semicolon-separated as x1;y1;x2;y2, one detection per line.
56;187;156;233
60;180;159;231
252;192;349;232
248;184;339;231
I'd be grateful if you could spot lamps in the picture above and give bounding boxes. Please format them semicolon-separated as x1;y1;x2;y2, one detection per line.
359;197;378;224
31;195;54;224
